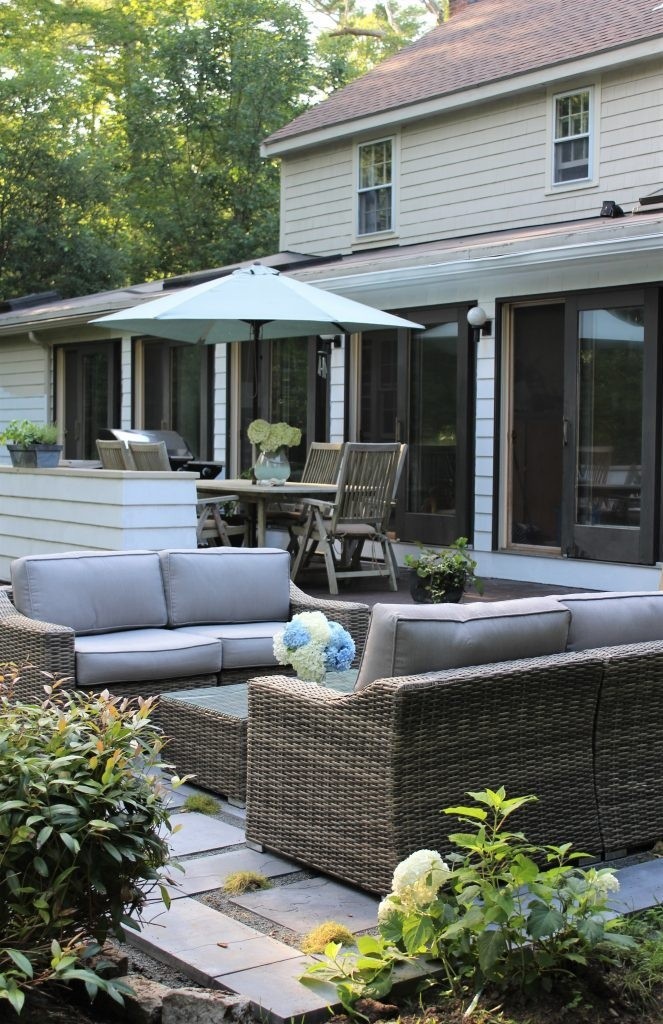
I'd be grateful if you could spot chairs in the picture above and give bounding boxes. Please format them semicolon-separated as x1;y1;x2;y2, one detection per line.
265;441;408;595
96;439;249;548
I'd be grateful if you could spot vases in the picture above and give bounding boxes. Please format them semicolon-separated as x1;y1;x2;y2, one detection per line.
254;445;290;485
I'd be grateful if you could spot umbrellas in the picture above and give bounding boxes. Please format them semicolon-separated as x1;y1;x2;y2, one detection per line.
85;262;426;547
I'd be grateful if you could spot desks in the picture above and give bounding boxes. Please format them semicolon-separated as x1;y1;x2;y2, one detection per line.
196;479;384;547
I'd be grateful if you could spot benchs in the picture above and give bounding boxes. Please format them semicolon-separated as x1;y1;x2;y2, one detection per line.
0;545;370;740
244;590;663;899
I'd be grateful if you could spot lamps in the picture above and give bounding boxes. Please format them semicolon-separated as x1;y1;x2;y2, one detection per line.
320;334;341;354
467;307;492;341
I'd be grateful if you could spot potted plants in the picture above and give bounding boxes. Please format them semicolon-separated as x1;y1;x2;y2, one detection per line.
404;536;484;604
0;420;64;468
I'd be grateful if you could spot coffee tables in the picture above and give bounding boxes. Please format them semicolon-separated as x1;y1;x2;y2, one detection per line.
160;669;358;809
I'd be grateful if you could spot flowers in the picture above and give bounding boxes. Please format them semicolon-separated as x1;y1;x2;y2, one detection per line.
248;419;301;452
273;610;355;682
